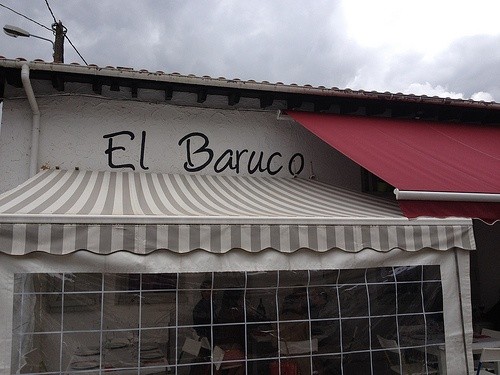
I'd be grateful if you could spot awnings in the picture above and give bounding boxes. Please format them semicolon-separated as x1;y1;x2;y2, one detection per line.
288;110;499;226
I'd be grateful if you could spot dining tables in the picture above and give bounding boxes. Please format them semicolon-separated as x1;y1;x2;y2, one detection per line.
399;332;500;375
248;322;328;356
62;342;171;375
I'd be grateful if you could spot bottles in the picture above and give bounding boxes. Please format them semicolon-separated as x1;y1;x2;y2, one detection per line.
256;298;266;321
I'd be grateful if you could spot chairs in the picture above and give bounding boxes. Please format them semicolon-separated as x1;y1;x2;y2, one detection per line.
473;348;500;375
171;328;248;375
15;347;50;375
376;333;439;375
396;325;438;365
278;337;320;375
311;326;358;375
474;328;500;373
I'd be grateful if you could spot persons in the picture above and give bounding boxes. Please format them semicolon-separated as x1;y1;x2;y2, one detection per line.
193;279;419;375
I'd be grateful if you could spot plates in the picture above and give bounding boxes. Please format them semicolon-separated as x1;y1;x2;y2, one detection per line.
72;337;159;357
410;334;432;341
137;351;164;360
71;361;100;370
